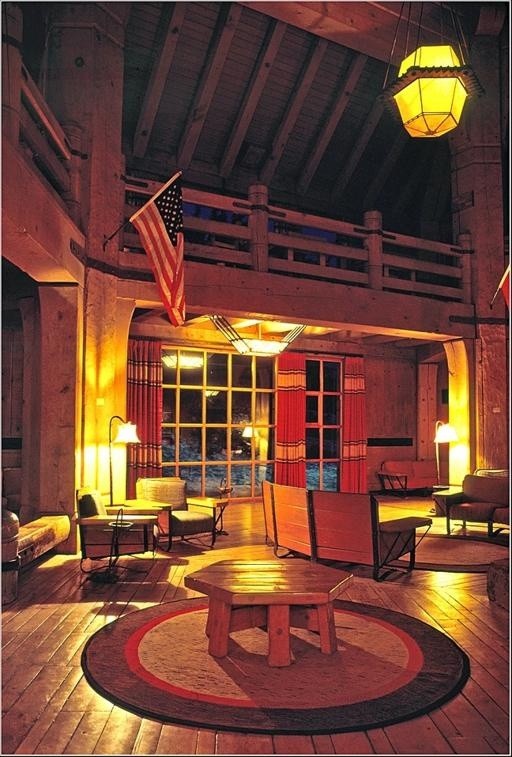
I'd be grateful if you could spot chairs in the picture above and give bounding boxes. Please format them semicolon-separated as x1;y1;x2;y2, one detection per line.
262;480;434;582
445;468;510;538
135;475;216;552
75;485;159;575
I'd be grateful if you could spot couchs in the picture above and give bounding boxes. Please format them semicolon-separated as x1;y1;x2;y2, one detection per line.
377;459;436;498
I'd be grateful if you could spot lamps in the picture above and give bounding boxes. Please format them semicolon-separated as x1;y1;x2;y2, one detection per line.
108;414;142;505
241;424;258;438
162;351;215;368
433;420;459;485
203;389;221;397
207;313;309;358
377;1;485;139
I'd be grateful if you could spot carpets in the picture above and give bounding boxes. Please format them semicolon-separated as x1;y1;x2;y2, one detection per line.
385;533;509;574
81;597;473;736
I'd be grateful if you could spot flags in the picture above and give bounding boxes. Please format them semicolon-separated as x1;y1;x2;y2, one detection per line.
500;273;510;307
131;181;186;329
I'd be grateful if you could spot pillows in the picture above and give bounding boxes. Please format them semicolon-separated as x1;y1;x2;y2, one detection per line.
461;473;508;504
146;479;189;511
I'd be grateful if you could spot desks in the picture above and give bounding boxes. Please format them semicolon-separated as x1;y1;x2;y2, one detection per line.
184;557;358;669
106;506;163;515
188;494;230;535
429;485;464;517
